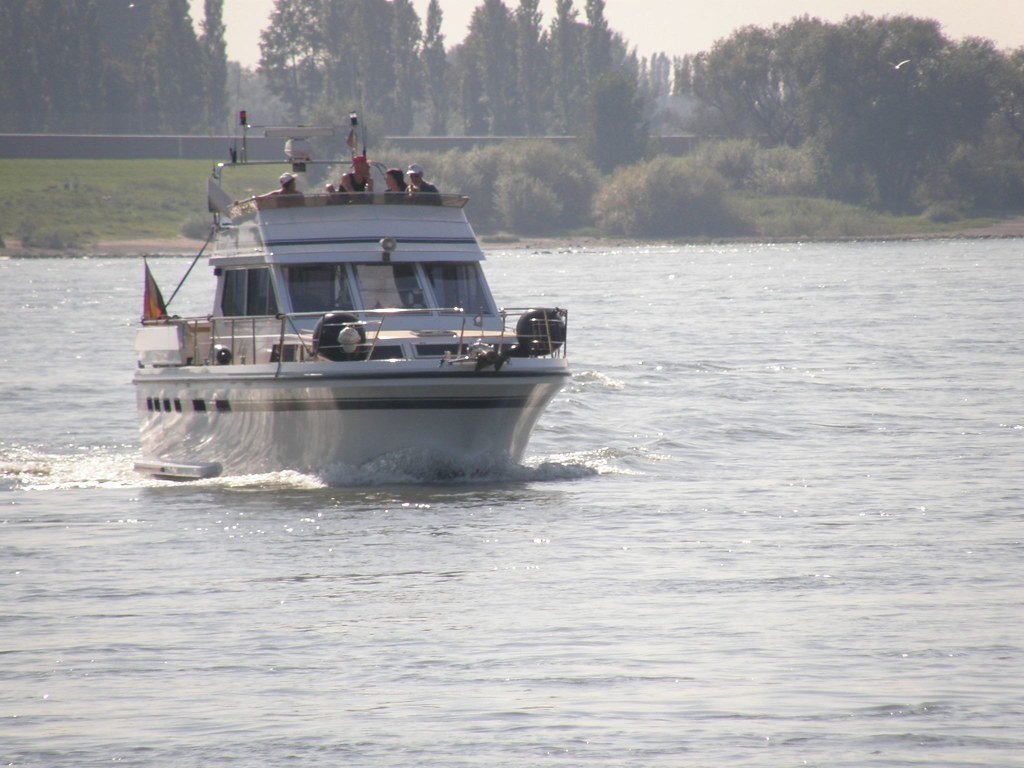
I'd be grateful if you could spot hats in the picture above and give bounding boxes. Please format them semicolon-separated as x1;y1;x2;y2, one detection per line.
406;164;423;174
280;173;297;185
350;155;364;168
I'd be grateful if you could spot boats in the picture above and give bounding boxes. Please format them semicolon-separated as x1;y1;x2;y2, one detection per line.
131;111;571;491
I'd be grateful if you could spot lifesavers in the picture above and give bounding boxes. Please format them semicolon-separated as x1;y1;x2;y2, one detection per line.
517;307;566;356
313;310;367;363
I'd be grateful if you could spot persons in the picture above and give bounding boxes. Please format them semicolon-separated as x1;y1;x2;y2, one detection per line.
259;172;304;208
384;167;408;204
339;155;375;205
403;163;442;206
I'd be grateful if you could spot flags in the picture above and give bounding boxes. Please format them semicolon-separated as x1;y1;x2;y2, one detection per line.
140;265;167;326
